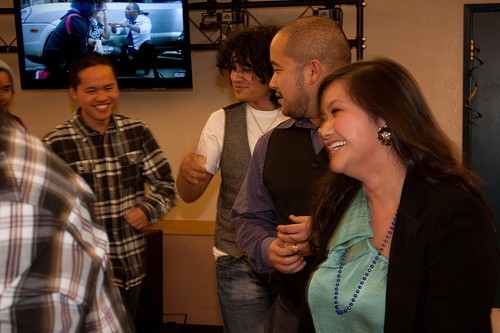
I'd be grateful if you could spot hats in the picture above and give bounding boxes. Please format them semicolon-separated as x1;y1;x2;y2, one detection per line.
0;60;16;94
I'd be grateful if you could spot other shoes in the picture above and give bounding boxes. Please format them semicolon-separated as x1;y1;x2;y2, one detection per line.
36;70;49;79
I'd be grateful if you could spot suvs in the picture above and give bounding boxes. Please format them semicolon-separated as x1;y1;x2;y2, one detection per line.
22;3;185;78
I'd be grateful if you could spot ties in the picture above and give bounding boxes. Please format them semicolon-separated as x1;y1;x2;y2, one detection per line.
127;22;136;55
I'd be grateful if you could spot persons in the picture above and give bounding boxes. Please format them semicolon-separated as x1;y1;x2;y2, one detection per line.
230;16;351;333
298;59;500;333
39;0;154;78
176;25;291;333
0;52;177;333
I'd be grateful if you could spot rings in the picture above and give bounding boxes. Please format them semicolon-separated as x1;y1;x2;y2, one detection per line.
293;243;297;247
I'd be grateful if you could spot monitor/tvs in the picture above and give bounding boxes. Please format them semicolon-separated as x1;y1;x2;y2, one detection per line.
12;0;192;89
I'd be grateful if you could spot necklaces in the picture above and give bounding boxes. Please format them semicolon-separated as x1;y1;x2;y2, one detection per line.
245;103;281;134
334;213;397;314
